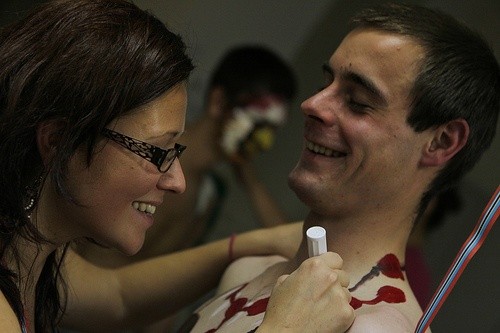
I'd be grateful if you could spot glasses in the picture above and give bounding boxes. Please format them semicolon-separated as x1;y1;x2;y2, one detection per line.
101;127;188;173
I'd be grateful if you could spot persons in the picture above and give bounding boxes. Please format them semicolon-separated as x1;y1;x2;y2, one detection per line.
226;137;464;313
74;43;297;333
179;2;500;333
0;0;357;333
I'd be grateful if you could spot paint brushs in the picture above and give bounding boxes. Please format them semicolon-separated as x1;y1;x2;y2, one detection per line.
306;224;329;260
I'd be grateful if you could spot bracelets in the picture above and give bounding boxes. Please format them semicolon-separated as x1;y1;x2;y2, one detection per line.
228;235;236;264
